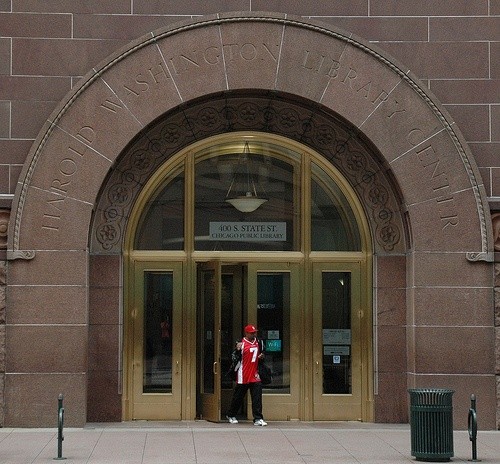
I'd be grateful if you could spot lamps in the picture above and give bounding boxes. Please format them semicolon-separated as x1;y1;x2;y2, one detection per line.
223;141;270;214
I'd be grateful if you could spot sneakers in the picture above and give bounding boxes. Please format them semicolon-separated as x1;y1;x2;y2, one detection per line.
228;417;238;424
253;419;267;426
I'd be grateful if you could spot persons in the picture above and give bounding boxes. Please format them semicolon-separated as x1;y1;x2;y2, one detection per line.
226;325;268;426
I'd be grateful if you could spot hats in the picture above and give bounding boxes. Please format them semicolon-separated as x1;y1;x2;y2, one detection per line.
244;324;258;333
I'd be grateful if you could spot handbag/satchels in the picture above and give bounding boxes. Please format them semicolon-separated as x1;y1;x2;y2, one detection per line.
258;363;272;385
